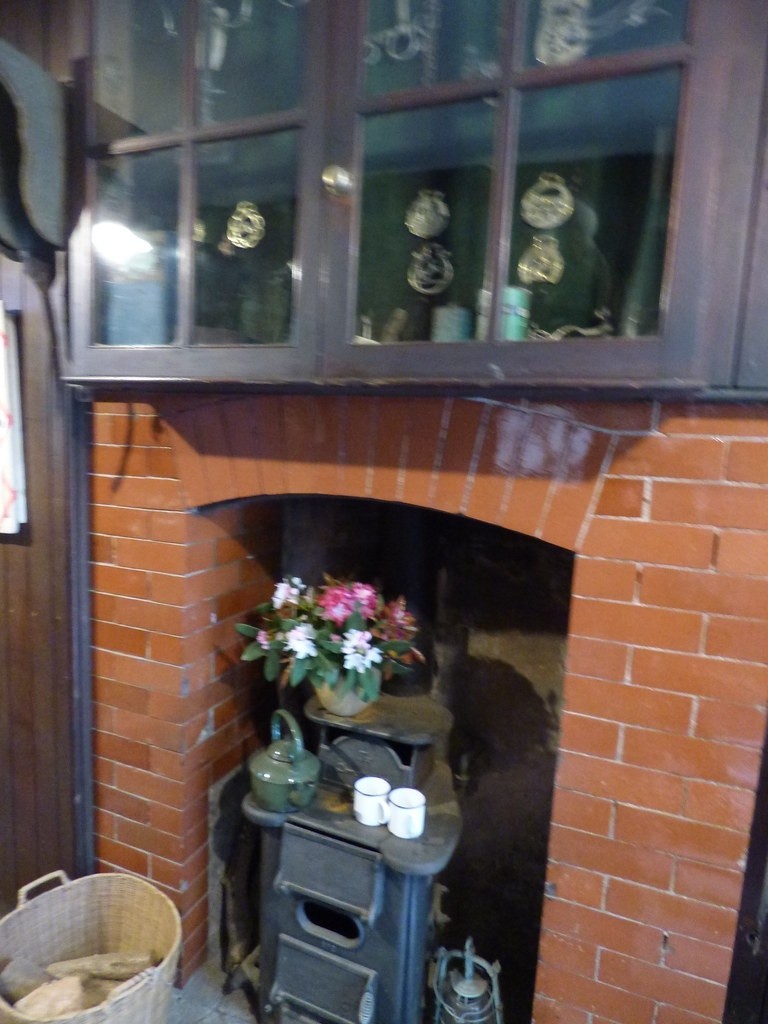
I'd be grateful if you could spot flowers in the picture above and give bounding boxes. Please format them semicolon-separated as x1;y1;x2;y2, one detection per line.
235;573;425;702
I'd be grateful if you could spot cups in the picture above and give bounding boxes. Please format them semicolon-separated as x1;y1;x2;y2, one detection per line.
352;776;391;827
503;286;532;340
430;306;473;342
387;787;427;839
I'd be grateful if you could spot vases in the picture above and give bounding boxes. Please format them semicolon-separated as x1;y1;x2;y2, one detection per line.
314;667;381;716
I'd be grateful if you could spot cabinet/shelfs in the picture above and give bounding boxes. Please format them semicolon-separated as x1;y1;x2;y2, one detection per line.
59;0;767;405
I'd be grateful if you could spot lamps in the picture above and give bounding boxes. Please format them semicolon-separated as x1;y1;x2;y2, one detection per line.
433;936;504;1024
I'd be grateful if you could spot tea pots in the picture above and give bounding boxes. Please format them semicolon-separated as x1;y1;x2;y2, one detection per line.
246;708;321;813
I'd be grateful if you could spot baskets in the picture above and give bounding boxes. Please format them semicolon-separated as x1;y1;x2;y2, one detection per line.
0;870;182;1024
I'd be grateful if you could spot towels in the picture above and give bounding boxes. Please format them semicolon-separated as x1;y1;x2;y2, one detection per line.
0;301;28;534
